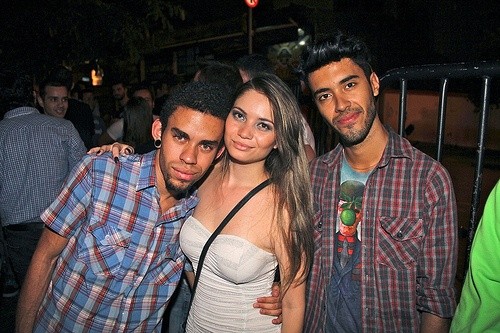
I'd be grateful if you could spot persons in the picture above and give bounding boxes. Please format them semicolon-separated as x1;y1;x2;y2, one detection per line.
84;70;317;333
295;28;461;329
447;179;500;333
17;79;285;332
0;62;244;333
235;53;317;160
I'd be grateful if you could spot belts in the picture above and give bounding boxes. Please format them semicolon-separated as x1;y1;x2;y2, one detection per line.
6;222;45;231
